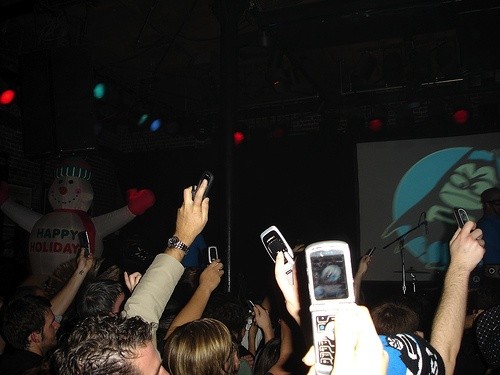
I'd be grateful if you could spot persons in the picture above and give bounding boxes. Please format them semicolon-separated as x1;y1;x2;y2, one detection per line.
0;179;500;375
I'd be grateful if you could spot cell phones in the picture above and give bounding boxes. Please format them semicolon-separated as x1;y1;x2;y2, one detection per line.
305;240;355;373
78;230;90;257
207;246;217;265
454;207;473;233
367;247;375;258
245;299;256;313
260;225;295;285
192;171;212;204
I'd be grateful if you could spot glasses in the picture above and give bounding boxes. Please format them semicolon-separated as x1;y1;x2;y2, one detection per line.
486;200;500;205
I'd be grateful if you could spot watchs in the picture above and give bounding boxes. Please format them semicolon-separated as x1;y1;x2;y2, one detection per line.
168;236;190;255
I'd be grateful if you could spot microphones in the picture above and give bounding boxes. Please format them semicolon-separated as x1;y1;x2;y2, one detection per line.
424;211;428;234
